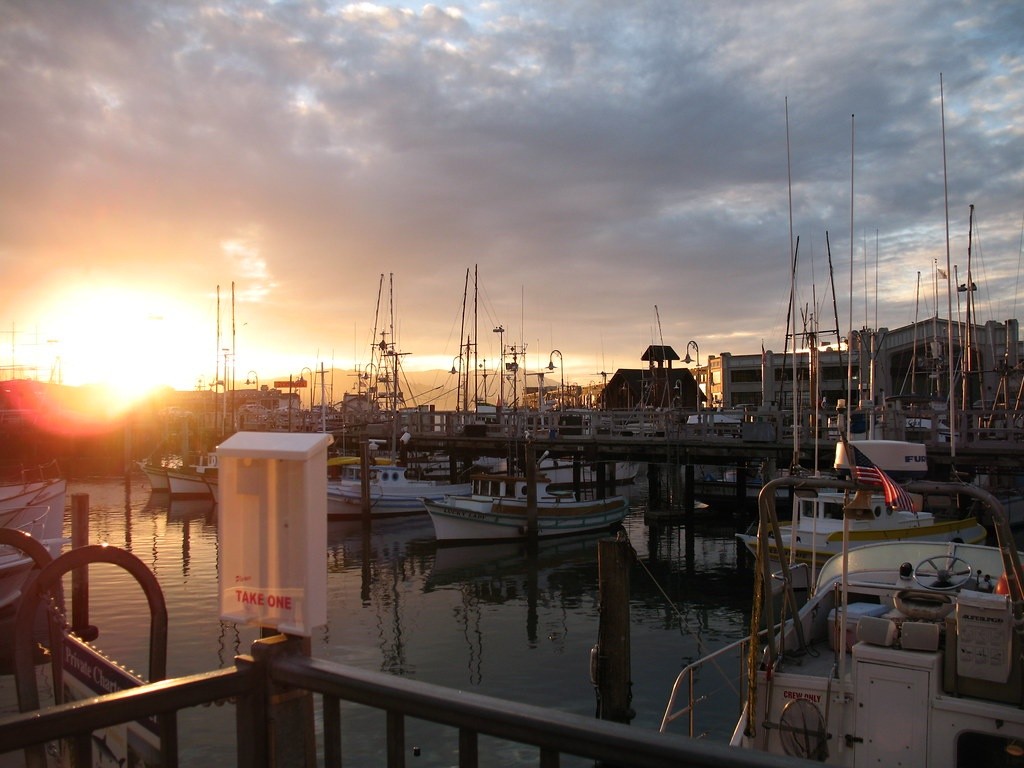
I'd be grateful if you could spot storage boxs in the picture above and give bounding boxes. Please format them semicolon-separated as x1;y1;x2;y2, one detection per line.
827;602;888;652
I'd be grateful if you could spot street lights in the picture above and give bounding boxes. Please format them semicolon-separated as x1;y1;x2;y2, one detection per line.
679;340;704;435
362;362;378;408
244;370;259;420
449;356;466;410
296;367;313;411
545;350;565;412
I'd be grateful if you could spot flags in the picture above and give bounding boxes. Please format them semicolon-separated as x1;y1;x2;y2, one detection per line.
849;442;916;514
762;345;767;364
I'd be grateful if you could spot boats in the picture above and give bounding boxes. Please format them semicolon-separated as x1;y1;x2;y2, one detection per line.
1;455;71;568
0;505;72;614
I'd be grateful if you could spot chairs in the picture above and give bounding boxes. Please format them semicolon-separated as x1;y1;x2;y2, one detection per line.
894;586;960;617
945;589;1024;709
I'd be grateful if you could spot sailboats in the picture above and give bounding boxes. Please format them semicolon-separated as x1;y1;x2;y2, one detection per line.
129;71;1024;768
